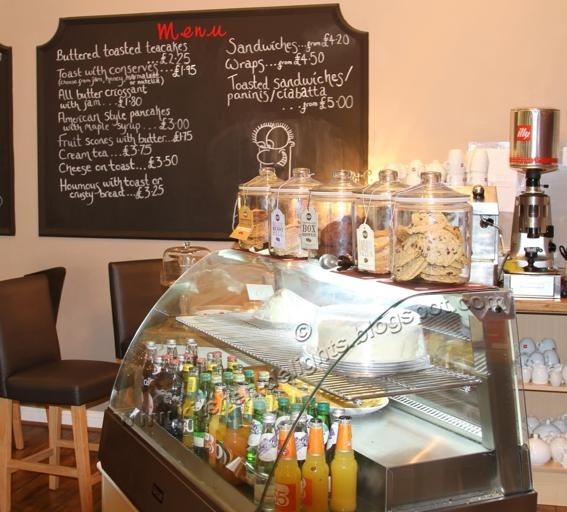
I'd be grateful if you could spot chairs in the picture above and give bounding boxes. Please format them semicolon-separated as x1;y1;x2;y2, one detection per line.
1;274;135;512
109;257;181;360
10;267;66;452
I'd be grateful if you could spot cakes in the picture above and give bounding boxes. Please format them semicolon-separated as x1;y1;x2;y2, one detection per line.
259;288;319;320
314;303;420;360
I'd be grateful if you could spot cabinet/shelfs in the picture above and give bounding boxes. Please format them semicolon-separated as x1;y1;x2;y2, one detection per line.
515;297;567;506
96;247;537;512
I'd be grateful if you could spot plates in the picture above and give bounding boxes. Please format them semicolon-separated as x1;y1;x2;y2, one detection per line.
289;371;388;419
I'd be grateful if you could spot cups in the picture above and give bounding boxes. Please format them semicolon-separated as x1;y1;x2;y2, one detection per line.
510;335;566;387
373;146;488;187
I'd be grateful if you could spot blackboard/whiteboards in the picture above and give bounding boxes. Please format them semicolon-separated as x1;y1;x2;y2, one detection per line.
35;3;372;239
0;47;15;235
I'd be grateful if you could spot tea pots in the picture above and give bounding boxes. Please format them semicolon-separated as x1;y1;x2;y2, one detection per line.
522;412;567;468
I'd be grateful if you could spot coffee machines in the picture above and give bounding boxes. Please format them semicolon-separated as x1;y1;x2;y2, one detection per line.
502;107;563;302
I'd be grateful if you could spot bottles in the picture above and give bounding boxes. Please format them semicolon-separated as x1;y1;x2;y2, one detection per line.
309;168;365;273
141;338;358;512
390;171;473;289
265;165;322;261
352;167;411;278
235;167;285;253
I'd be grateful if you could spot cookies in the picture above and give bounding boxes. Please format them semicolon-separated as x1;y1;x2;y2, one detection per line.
238;208;470;283
